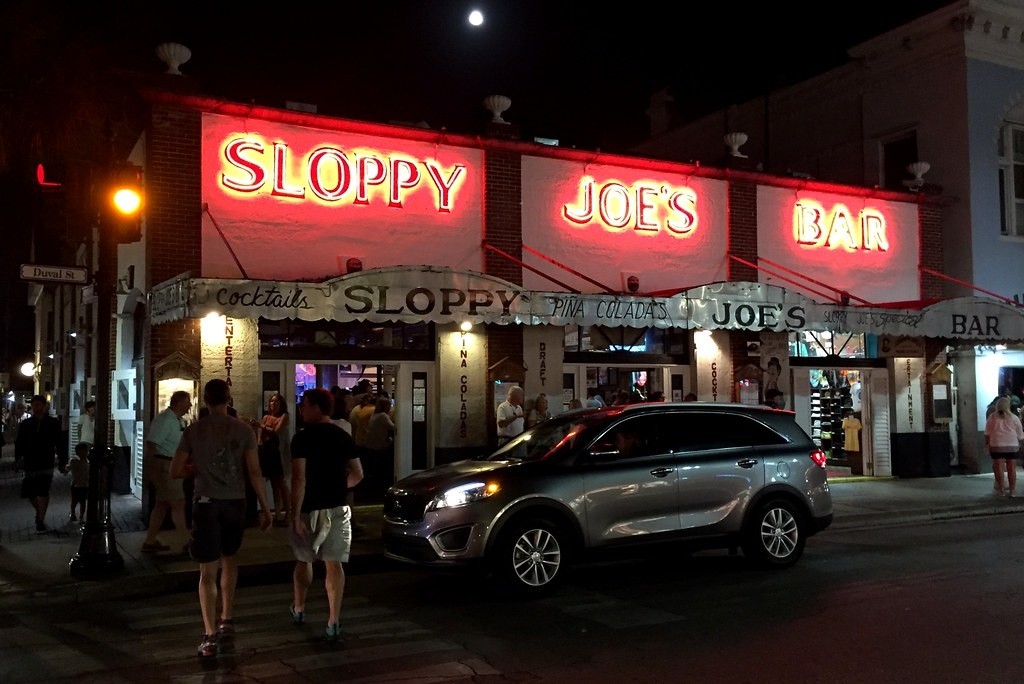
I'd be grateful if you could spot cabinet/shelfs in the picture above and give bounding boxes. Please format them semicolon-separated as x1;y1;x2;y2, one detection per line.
810;388;853;466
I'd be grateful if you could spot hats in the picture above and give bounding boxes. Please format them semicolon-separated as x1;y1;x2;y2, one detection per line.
31;395;46;404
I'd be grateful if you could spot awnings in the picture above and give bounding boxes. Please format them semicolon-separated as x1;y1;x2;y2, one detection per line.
148;265;1024;342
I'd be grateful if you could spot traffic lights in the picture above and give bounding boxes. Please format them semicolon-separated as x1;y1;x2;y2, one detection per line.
109;163;141;242
36;158;67;187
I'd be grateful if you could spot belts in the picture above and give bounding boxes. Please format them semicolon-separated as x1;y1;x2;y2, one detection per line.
152;453;173;461
498;436;512;439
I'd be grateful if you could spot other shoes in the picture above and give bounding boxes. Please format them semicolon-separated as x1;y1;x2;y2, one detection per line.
182;538;194;551
351;519;368;536
198;634;217;660
286;598;305;627
141;540;170;553
273;512;281;526
80;520;85;524
994;482;1009;491
218;617;235;652
310;621;342;642
1009;490;1021;498
996;490;1005;497
279;516;290;530
71;515;77;521
34;522;48;534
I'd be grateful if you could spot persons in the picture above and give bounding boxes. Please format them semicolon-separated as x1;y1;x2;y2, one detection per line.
12;380;397;553
983;385;1024;497
495;379;697;450
169;379;272;657
287;387;364;640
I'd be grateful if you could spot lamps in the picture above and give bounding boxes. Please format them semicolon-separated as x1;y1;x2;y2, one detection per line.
21;360;51;377
48;352;70;359
459;320;473;334
68;327;94;339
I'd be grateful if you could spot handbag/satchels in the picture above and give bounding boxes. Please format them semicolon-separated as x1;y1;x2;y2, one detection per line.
257;424;285;481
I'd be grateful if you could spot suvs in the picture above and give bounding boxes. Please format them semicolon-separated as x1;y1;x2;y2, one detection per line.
384;403;834;592
